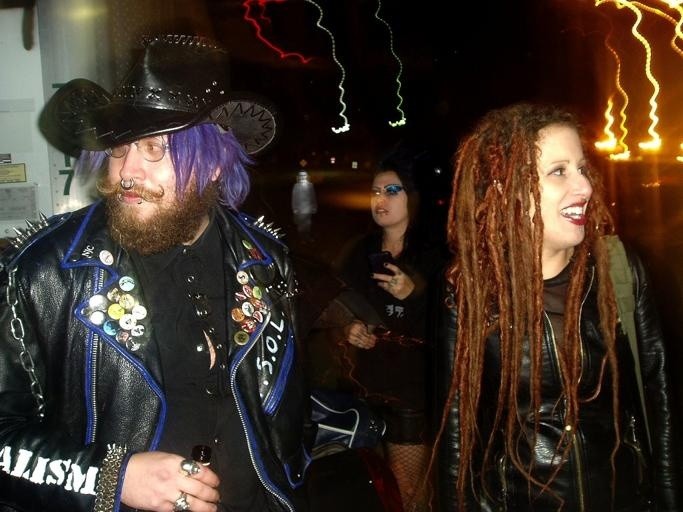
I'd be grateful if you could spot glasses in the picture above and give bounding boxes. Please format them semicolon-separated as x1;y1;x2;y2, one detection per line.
104;134;170;163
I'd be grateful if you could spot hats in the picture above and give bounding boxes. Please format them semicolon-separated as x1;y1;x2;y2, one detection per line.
47;29;277;157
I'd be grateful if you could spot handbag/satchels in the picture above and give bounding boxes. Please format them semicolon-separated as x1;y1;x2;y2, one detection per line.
301;386;383;451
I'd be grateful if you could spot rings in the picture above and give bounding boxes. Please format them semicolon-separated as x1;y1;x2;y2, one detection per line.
172;492;190;512
179;459;202;476
388;276;398;287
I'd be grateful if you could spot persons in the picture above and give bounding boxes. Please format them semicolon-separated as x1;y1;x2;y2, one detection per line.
0;26;396;512
421;101;681;512
308;157;448;512
